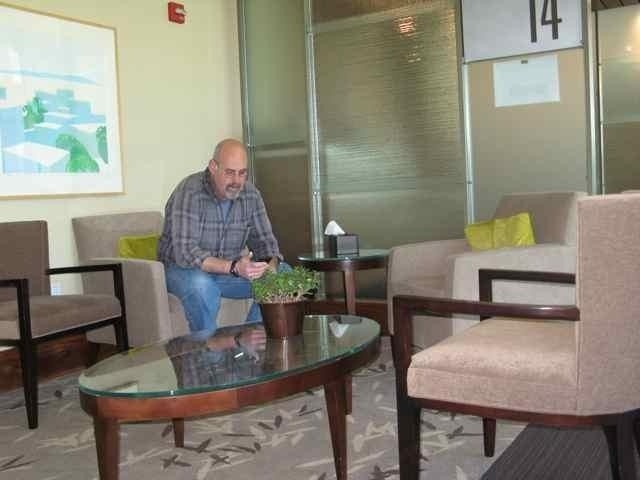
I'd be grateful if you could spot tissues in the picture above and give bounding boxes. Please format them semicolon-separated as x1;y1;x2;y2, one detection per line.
325;219;359;256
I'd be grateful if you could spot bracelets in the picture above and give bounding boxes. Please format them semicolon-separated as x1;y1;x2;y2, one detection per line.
233;332;242;348
230;258;242;279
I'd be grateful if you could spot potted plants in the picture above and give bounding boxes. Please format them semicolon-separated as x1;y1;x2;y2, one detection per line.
245;262;322;338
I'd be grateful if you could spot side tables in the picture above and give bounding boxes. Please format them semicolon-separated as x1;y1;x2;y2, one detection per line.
299;248;391;317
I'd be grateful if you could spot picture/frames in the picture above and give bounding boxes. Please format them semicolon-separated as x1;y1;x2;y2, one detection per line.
0;1;127;201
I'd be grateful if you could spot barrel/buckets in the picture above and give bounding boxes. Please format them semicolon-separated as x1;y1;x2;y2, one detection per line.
257;297;311;338
262;338;307;372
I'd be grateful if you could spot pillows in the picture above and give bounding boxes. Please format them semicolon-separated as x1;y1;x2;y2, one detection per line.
115;234;162;263
465;212;535;252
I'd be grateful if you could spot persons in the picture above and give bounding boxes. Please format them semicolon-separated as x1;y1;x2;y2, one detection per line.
166;321;268;391
156;140;293;332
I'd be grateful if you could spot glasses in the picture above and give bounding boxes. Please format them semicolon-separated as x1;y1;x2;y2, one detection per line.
211;160;247;177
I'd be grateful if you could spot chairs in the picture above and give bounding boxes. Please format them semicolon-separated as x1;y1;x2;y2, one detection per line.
390;188;589;350
385;188;640;479
0;220;129;430
71;211;255;369
